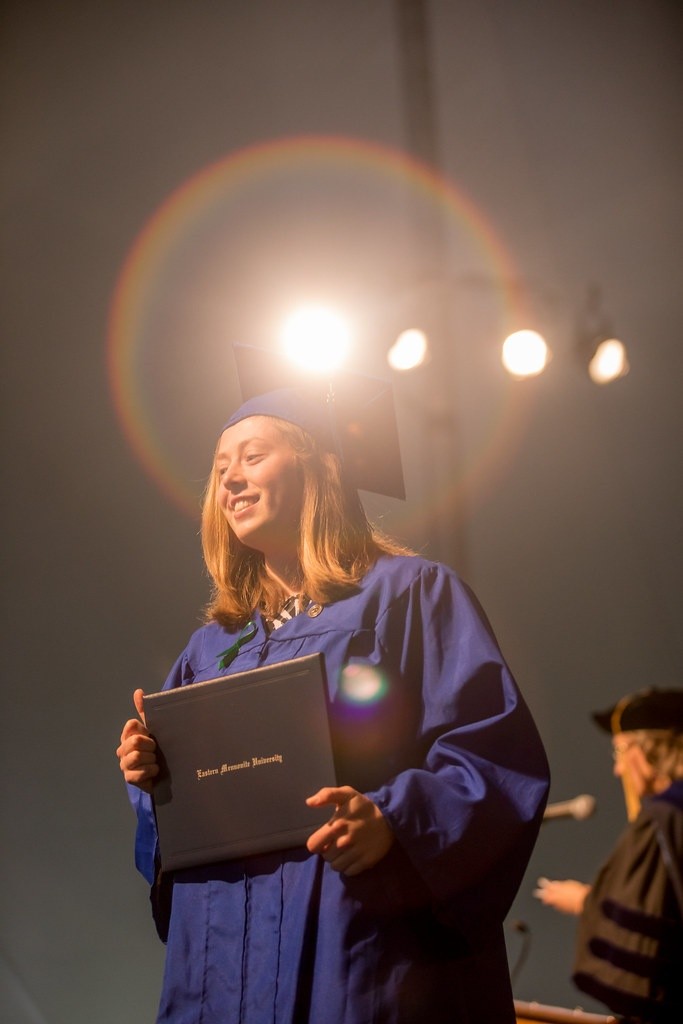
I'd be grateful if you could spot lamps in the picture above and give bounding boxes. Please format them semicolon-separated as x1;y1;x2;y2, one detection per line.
573;286;630;384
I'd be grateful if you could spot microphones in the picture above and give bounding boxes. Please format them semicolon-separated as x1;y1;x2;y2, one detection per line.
510;921;532;979
542;793;597;820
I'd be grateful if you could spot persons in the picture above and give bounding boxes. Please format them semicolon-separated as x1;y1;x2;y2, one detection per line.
534;683;683;1024
116;340;551;1024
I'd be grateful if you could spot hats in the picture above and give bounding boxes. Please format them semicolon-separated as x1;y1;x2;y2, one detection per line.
590;685;683;734
219;341;405;502
